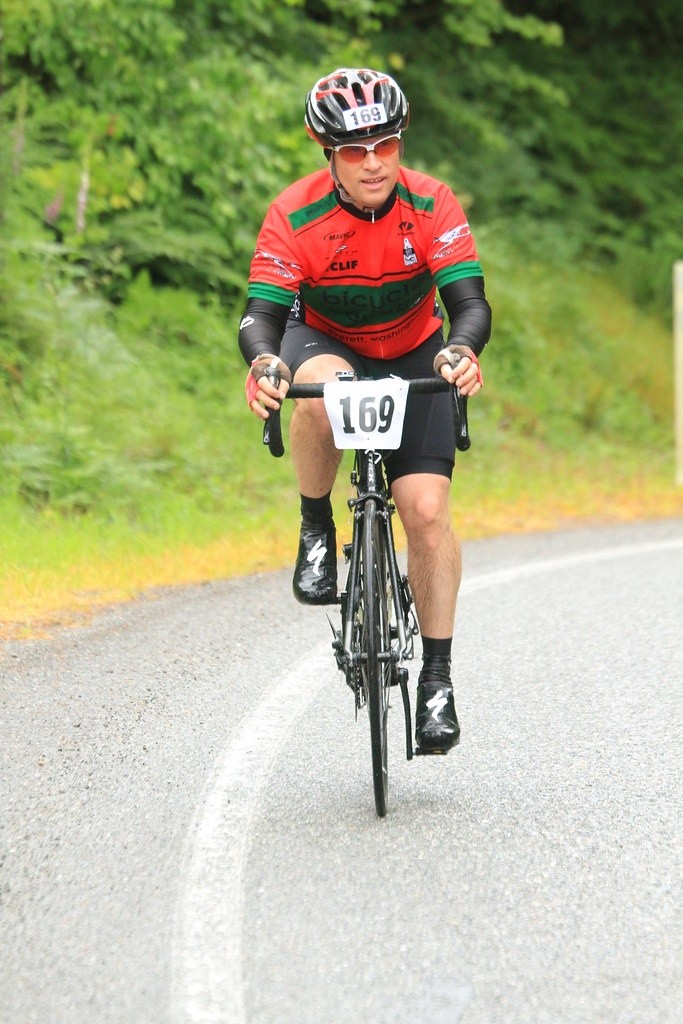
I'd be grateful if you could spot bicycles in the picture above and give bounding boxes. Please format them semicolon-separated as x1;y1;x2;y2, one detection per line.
260;355;473;818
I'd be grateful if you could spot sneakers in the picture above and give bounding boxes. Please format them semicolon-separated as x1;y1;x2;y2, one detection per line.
415;668;461;753
292;521;338;605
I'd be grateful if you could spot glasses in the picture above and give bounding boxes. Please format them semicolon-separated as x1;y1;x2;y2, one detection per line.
325;129;401;162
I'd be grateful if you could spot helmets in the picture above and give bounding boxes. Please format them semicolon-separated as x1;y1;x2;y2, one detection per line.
304;68;410;158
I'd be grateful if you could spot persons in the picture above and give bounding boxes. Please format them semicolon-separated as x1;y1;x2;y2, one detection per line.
237;68;493;751
